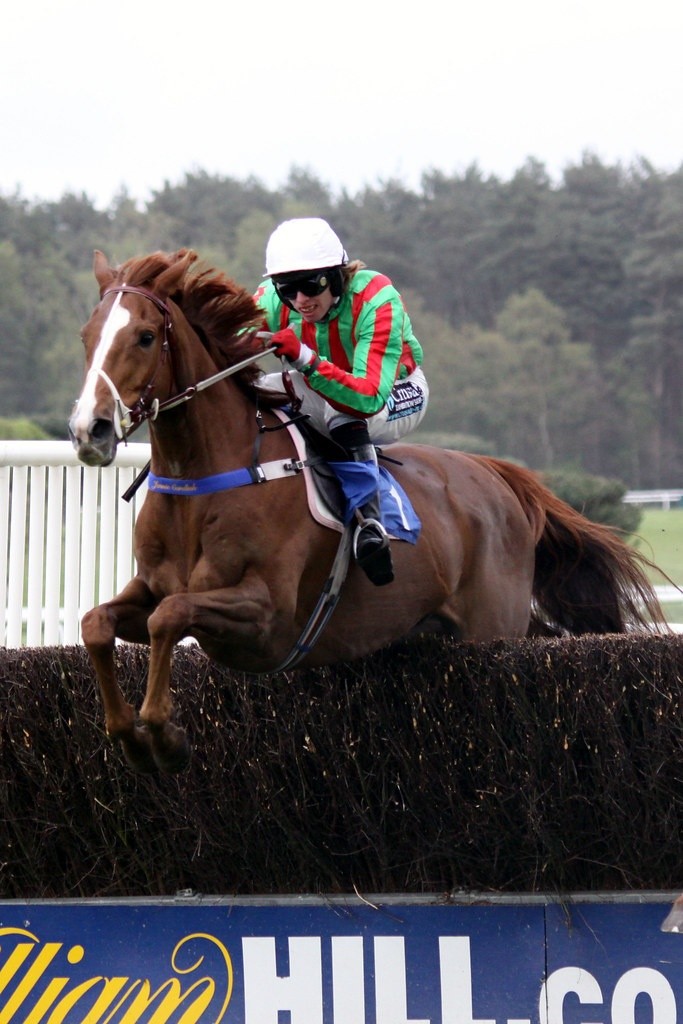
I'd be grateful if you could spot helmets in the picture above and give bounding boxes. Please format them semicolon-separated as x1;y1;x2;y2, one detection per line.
262;217;349;277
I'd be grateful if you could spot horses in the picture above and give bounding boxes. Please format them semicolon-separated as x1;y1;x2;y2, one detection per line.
67;248;683;775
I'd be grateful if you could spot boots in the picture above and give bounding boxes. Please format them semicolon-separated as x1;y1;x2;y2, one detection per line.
348;443;394;586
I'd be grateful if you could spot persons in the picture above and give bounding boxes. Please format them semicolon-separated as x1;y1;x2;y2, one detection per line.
237;217;430;587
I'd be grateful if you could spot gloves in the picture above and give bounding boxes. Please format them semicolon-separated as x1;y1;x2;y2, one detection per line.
265;329;301;363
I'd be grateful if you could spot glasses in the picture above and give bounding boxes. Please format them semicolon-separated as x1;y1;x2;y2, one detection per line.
276;273;329;299
